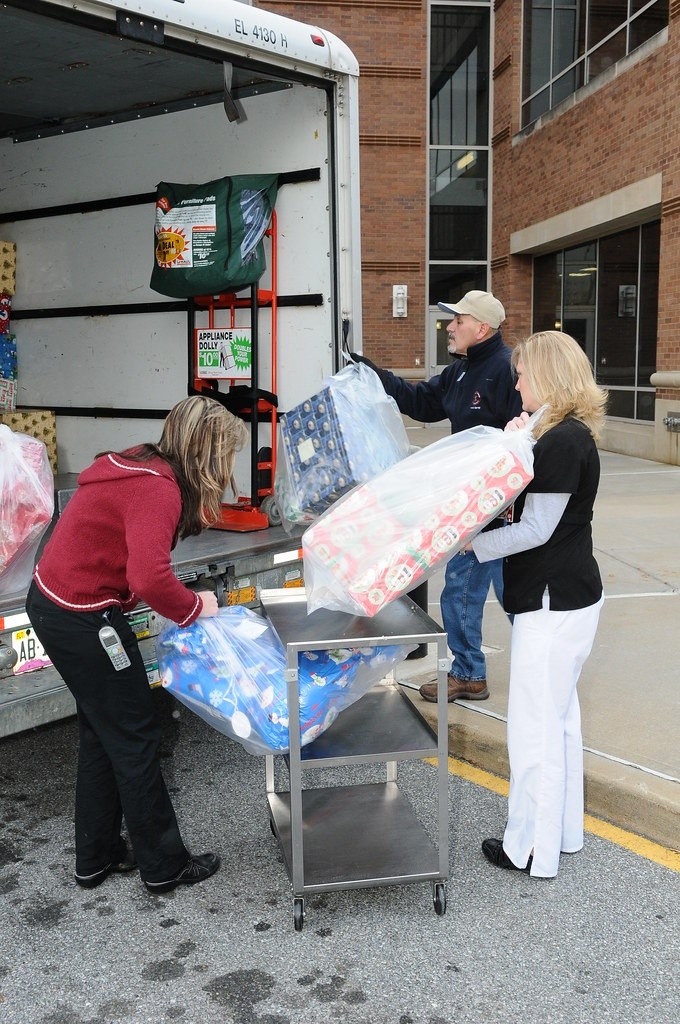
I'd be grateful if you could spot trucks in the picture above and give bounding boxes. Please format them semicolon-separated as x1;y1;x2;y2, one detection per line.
0;1;363;735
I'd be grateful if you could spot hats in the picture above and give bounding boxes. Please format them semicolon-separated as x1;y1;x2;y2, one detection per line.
438;289;506;329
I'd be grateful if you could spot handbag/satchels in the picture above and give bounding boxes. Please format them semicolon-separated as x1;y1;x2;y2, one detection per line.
150;172;279;298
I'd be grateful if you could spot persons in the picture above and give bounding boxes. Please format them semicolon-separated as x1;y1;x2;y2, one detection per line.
348;291;524;701
463;330;610;878
26;394;247;892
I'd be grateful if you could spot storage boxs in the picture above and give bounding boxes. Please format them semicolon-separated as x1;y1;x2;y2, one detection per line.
301;444;533;618
278;387;399;515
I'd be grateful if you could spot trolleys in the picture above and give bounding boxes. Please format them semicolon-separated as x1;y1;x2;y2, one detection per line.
254;592;453;930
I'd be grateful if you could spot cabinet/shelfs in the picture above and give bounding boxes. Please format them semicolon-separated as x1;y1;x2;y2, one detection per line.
258;586;454;934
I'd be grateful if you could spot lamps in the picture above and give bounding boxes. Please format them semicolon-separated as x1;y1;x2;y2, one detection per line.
394;287;408;316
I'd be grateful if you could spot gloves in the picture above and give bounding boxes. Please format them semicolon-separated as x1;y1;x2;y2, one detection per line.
345;352;390;391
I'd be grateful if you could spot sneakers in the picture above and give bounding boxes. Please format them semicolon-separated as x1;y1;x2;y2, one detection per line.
74;850;140;888
419;673;491;703
145;851;220;893
482;838;533;871
